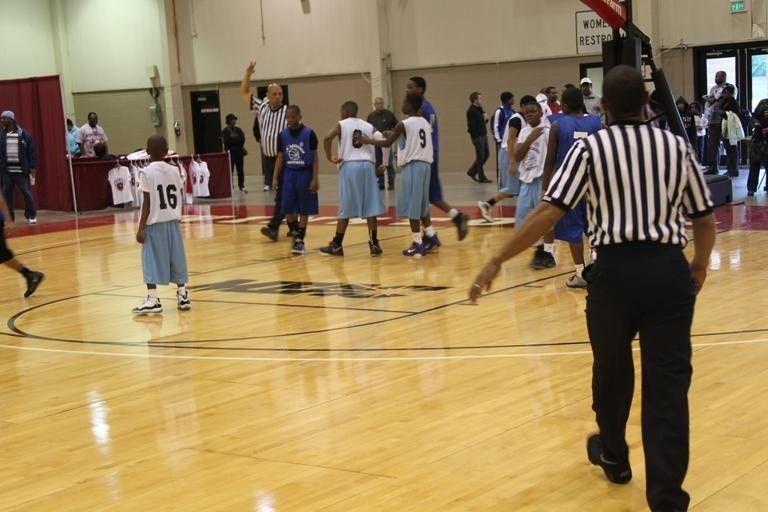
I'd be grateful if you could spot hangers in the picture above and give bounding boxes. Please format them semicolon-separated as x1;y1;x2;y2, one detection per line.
114;153;205;170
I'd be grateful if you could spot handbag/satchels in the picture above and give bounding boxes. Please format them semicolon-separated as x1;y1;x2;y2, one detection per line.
94;142;108;156
243;148;248;155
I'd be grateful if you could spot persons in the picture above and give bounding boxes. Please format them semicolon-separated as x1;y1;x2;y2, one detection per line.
0;193;45;298
241;60;320;255
320;76;468;256
93;143;118;160
75;112;109;157
1;110;37;223
131;135;192;316
465;77;604;287
67;119;83;157
469;63;717;511
221;113;247;191
647;70;768;195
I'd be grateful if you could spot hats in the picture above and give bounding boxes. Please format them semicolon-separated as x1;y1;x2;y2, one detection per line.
225;114;237;124
0;111;17;122
25;216;38;224
21;271;43;298
580;77;592;85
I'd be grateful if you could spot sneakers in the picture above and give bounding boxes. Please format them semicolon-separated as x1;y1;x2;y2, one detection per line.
529;249;556;269
368;239;383;257
452;211;469;241
587;434;633;484
566;272;588;288
722;171;734;177
422;232;442;250
403;241;425;257
476;200;495;223
239;189;248;193
288;222;305;236
175;289;191;310
703;170;719;176
263;185;271;191
131;296;162;314
747;191;754;196
291;237;305;255
319;241;344;256
260;226;279;241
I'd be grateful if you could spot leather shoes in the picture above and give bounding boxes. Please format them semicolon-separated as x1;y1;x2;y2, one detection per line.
479;179;493;184
467;172;479;183
